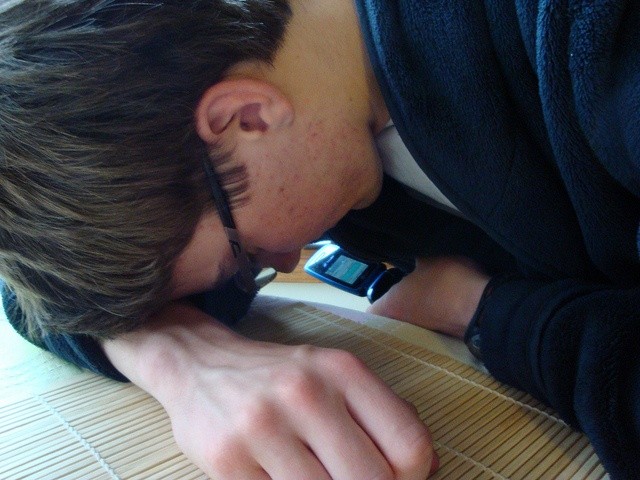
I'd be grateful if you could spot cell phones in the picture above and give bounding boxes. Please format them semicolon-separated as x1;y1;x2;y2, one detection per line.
304;243;408;304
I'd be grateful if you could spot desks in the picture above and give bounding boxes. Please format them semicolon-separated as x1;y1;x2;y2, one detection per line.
261;244;394;282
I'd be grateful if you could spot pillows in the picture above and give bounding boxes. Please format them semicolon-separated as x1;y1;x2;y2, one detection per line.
0;300;613;479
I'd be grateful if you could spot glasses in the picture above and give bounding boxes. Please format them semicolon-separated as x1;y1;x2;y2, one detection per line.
199;150;276;295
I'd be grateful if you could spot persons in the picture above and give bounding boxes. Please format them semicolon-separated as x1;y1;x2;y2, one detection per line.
0;0;640;480
